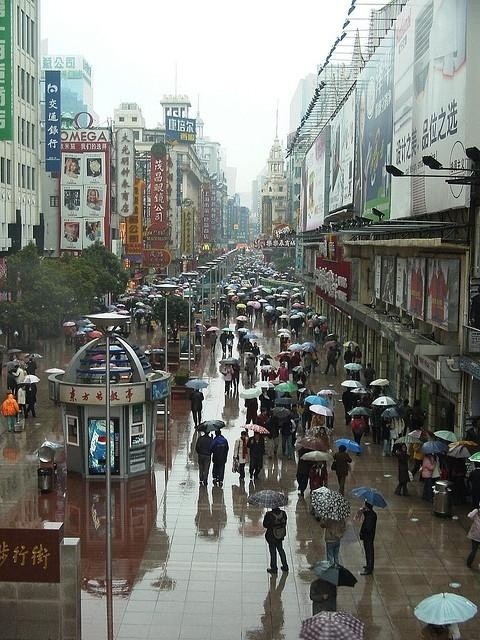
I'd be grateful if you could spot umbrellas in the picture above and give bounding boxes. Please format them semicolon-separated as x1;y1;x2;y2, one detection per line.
309;561;357;588
300;611;364;640
43;367;66;374
414;592;478;625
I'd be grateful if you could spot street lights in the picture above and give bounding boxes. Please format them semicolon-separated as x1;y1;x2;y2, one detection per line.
82;308;136;576
181;272;199;378
197;248;239;353
152;426;180;593
178;399;199;494
153;282;179;426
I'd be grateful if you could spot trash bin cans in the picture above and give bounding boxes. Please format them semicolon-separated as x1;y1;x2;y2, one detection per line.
38;467;53;493
433;480;451;517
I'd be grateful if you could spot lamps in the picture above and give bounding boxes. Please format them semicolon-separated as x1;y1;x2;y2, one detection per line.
312;208;386;235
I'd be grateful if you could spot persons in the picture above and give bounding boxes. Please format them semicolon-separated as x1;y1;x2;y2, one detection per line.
263;507;289;572
310;579;337;616
360;501;377;575
185;249;480;515
62;273;185;354
0;345;42;433
86;189;102;210
467;508;480;568
63;222;78;241
422;623;461;640
86;222;101;240
64;158;79;177
320;516;346;564
